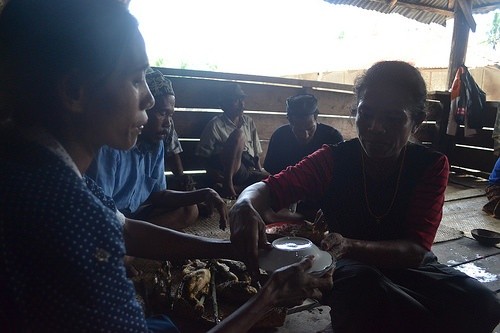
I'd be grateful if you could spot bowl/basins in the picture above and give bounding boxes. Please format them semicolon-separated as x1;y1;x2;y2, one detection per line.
259;235;332;274
471;228;500;246
265;222;307;241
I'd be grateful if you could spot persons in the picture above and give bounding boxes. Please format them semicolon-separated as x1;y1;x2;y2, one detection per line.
258;92;343;235
1;1;336;332
197;80;271;199
482;157;500;220
228;61;500;333
87;67;228;273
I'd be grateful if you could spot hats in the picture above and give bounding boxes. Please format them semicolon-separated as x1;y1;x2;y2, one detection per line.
144;71;176;95
215;83;248;99
285;93;318;115
